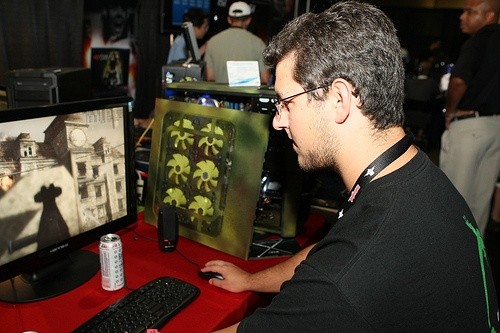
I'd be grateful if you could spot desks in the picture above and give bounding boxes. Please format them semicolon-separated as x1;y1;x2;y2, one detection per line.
0;206;339;333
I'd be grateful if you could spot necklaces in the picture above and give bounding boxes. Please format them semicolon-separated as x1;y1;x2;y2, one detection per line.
333;130;414;221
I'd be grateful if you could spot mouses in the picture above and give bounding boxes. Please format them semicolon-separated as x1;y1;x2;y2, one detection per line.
198;270;224;280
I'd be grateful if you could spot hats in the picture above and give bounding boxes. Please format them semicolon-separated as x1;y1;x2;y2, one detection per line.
229;1;251;17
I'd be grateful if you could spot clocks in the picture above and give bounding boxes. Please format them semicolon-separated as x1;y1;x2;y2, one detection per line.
70;127;88;147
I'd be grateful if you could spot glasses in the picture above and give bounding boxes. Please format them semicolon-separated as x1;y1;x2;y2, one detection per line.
271;83;331;123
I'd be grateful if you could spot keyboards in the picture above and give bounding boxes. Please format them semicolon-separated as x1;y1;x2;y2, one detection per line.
70;275;200;333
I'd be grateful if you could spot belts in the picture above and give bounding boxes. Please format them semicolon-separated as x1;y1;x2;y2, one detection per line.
448;112;493;122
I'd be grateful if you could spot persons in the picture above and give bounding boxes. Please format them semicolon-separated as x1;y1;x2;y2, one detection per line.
201;0;500;333
204;1;270;84
439;0;499;239
164;7;209;99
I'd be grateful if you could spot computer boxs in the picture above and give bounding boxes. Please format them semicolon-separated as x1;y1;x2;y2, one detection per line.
166;80;306;239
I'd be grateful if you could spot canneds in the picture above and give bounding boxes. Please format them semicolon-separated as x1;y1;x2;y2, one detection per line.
99;233;124;291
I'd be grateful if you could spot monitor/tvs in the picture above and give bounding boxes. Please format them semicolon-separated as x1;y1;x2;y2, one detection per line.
0;95;138;304
